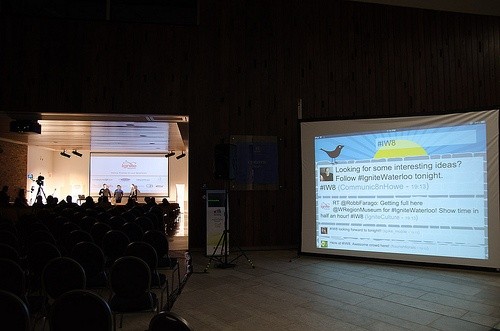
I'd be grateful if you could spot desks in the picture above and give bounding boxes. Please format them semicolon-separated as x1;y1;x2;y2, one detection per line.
77;199;86;205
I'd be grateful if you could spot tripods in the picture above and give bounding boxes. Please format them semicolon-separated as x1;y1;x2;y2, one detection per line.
204;182;255;273
35;186;47;203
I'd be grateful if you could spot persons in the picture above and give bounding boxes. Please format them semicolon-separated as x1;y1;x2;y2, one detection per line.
0;188;174;217
128;184;137;199
113;185;123;203
99;184;112;202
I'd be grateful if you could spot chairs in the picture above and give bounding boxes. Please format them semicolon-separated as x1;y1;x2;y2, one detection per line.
0;203;195;331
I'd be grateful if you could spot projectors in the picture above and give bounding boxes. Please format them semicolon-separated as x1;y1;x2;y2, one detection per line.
9;121;41;135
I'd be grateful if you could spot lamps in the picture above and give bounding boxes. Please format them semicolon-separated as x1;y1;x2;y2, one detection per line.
60;149;70;158
72;148;82;157
176;151;186;159
165;151;175;157
